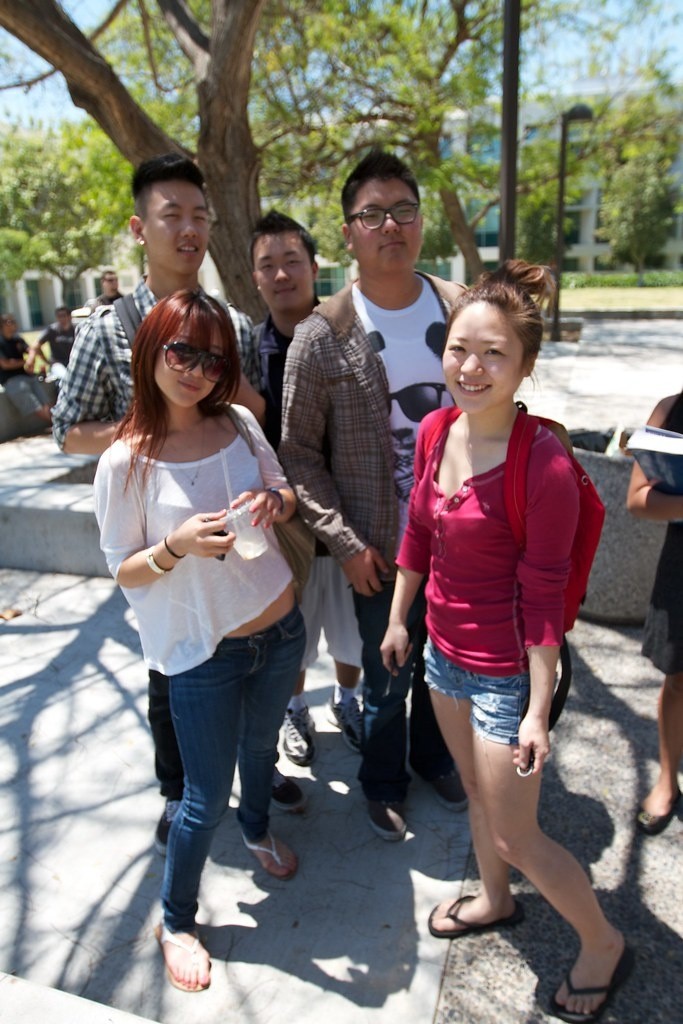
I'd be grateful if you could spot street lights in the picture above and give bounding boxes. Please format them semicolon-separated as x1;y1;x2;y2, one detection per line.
549;103;597;344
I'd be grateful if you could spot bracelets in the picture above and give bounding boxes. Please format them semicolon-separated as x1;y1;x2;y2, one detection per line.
267;487;284;515
164;534;188;559
145;545;175;576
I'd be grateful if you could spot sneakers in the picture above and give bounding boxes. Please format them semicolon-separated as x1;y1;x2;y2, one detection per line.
153;800;182;856
366;792;408;841
324;689;363;753
281;704;318;767
270;766;304;810
418;769;470;811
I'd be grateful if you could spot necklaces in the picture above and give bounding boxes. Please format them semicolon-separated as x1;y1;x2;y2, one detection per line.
161;416;205;486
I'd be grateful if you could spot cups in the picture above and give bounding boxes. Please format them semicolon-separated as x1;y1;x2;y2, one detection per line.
218;498;267;559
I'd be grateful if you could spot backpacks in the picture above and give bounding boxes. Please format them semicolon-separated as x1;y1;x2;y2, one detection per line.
424;403;607;608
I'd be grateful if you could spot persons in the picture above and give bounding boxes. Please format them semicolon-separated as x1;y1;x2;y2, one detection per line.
92;289;310;993
242;208;368;768
278;152;473;842
626;387;683;836
49;150;310;859
88;270;124;318
0;306;80;434
380;258;627;1024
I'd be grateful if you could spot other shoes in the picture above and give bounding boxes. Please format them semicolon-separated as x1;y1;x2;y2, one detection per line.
634;786;683;836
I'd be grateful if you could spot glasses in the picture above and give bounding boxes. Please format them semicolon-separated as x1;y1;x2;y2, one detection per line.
163;341;231;385
347;201;420;230
382;382;447;423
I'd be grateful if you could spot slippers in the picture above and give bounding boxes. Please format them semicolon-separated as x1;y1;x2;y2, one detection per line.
154;917;212;992
241;828;298;882
548;938;636;1023
427;893;527;938
